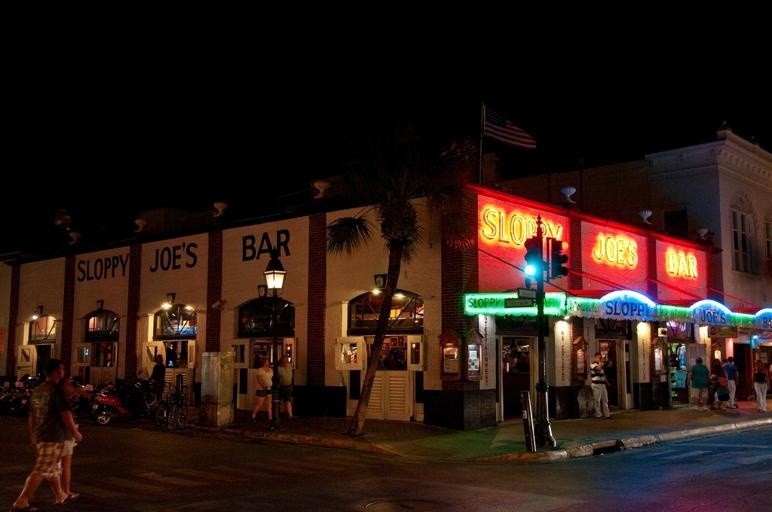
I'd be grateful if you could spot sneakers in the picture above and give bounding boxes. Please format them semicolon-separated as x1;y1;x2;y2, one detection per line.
758;409;767;415
265;420;274;425
595;415;604;419
690;403;739;412
605;417;615;420
288;416;297;421
250;416;257;425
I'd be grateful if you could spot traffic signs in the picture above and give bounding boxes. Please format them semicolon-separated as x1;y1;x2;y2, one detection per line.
504;297;534;309
517;288;536;298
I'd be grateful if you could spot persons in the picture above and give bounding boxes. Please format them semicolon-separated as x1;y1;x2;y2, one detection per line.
63;435;81;500
11;358;86;512
687;354;739;412
248;357;276;429
749;358;770;414
277;354;298;422
589;352;616;420
151;355;166;402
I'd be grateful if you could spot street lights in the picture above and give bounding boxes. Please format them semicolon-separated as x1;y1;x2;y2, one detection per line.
262;244;287;428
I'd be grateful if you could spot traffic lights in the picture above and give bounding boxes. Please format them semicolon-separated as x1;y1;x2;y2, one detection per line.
550;239;570;279
524;237;540;280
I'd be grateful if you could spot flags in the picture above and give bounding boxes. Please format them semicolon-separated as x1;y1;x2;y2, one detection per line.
482;103;537;149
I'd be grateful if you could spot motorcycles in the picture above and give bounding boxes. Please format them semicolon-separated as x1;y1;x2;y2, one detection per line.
0;370;159;426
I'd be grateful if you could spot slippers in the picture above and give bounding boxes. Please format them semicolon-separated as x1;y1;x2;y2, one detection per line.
53;492;79;505
10;503;40;512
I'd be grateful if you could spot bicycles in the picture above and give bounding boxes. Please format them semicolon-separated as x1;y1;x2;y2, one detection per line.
152;378;190;435
